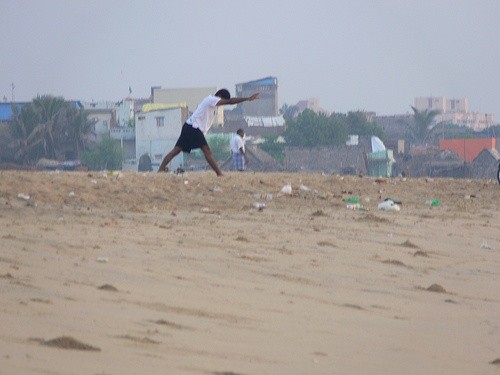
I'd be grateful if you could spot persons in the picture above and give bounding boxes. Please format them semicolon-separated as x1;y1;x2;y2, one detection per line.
231;127;246;172
156;89;262;176
